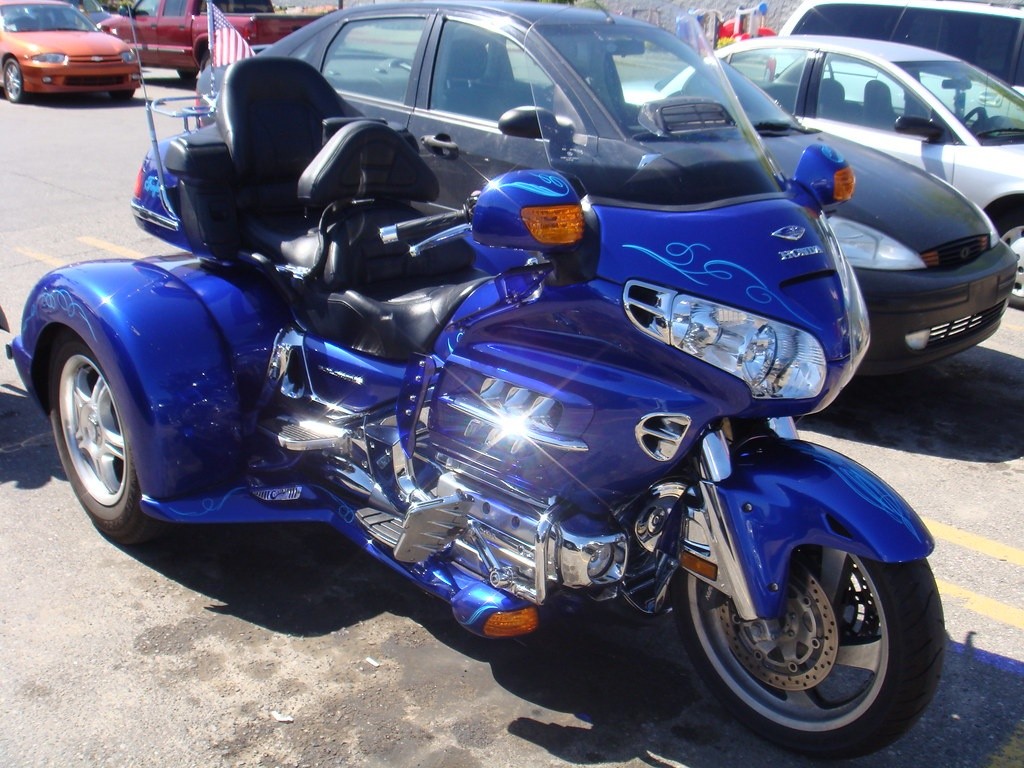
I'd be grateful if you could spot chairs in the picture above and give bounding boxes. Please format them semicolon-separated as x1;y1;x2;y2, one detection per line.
819;78;861;124
213;56;365;263
445;40;515;120
863;80;898;130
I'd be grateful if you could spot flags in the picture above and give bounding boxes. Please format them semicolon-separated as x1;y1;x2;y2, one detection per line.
207;0;256;67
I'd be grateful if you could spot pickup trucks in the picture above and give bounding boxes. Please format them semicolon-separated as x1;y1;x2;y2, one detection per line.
96;0;331;83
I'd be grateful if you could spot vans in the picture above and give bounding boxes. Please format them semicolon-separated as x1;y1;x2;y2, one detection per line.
763;0;1024;133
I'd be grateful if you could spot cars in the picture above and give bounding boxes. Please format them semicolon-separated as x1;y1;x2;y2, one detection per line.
62;0;111;25
188;1;1021;378
623;32;1024;311
0;0;142;105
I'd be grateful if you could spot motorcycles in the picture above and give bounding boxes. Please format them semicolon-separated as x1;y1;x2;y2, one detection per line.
6;0;949;762
